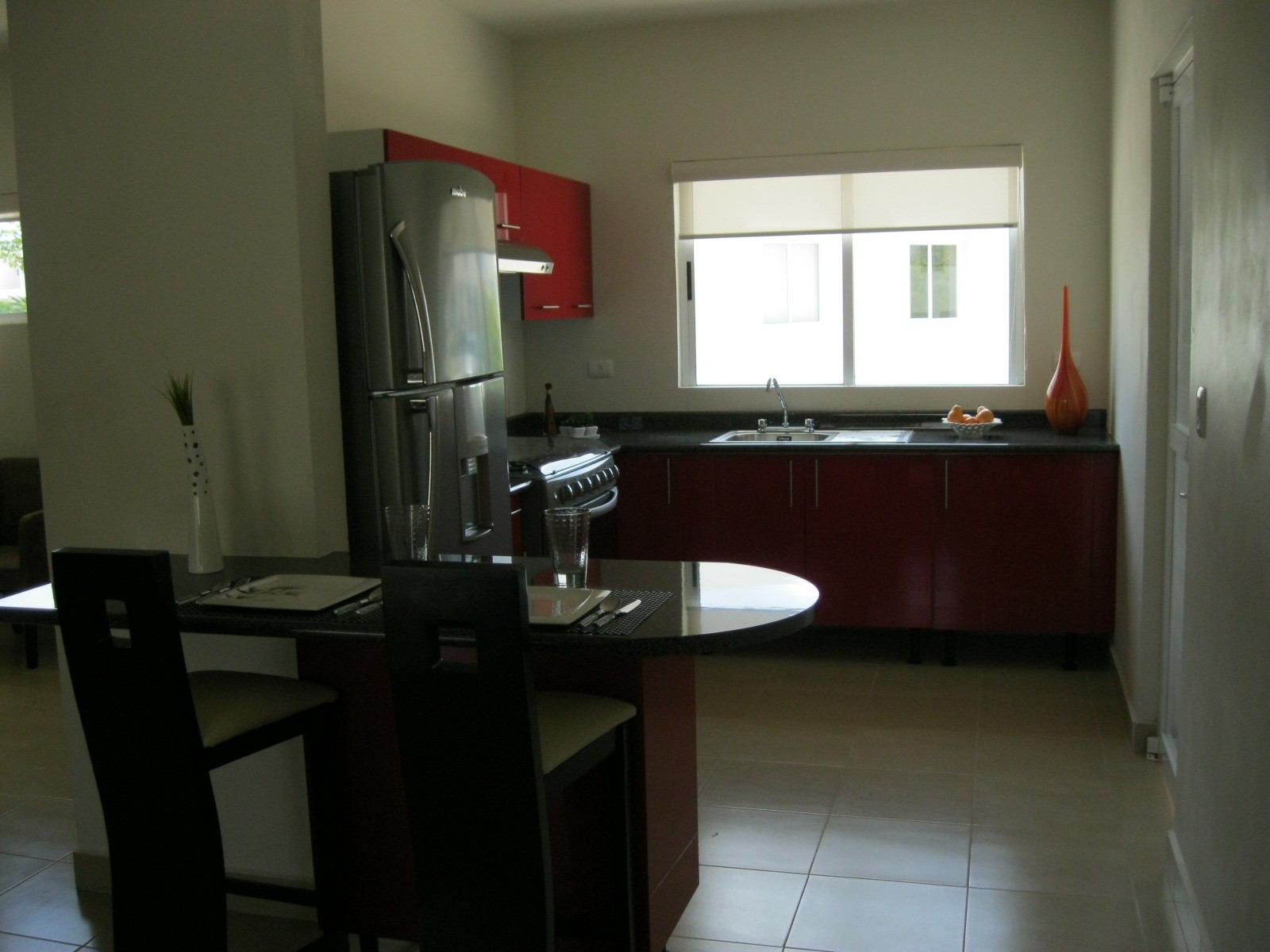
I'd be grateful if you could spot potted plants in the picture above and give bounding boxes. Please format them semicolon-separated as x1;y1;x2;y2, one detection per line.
559;412;599;436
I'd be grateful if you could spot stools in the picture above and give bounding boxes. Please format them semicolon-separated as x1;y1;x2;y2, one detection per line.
378;559;640;952
52;550;369;951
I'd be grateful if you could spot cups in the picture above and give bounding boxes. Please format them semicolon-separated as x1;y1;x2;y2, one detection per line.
559;426;572;436
544;507;591;588
570;427;585;437
385;505;431;561
583;426;598;436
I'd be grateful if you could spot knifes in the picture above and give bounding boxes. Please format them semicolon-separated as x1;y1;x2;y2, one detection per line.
594;599;641;627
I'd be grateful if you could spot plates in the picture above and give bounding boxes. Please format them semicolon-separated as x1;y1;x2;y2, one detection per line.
527;586;611;623
196;574;382;611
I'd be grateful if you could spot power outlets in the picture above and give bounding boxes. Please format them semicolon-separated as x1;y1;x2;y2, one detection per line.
588;359;613;379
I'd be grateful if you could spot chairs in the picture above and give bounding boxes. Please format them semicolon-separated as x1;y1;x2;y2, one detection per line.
0;457;50;670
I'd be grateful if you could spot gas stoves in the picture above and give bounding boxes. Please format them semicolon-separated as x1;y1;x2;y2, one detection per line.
507;436;619;509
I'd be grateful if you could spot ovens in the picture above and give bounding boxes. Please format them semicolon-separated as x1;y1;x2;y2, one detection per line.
531;486;619;558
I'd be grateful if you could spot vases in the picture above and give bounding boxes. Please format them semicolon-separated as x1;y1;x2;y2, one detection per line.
181;424;223;574
1045;285;1093;427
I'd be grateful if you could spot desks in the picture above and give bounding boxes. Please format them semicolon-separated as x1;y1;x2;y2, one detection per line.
0;551;819;952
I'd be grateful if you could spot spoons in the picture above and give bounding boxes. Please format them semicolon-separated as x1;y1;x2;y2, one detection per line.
334;587;381;615
195;575;250;603
579;596;620;627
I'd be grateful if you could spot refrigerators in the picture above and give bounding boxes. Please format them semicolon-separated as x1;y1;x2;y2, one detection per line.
330;159;512;555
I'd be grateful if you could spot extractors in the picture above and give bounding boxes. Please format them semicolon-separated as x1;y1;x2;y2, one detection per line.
497;223;554;274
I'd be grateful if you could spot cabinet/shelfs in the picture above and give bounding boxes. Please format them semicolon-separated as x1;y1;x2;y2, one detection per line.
327;129;521;244
715;453;933;664
500;166;594;322
932;455;1117;670
613;453;715;561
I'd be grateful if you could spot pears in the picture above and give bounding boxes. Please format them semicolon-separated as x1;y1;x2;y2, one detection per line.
947;404;994;424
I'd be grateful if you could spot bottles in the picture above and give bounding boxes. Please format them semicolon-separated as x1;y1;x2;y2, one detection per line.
1045;285;1088;436
544;383;559;437
